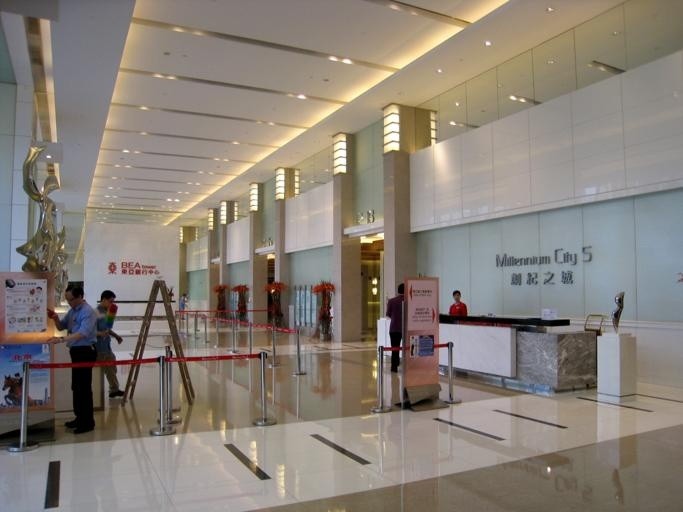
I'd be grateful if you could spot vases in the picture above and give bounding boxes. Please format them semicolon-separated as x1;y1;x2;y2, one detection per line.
320;333;331;341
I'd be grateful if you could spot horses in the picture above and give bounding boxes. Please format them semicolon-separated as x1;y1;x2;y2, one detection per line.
2;374;36;406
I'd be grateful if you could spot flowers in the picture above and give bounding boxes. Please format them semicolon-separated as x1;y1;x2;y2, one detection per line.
264;283;283;328
232;285;249;317
314;281;335;334
214;285;227;310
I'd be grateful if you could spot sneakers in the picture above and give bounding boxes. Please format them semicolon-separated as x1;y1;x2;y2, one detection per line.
64;388;125;435
391;366;399;372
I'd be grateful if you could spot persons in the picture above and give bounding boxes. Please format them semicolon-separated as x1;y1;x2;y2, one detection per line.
179;292;186;319
447;290;467;314
89;290;126;398
385;283;404;372
45;281;97;434
